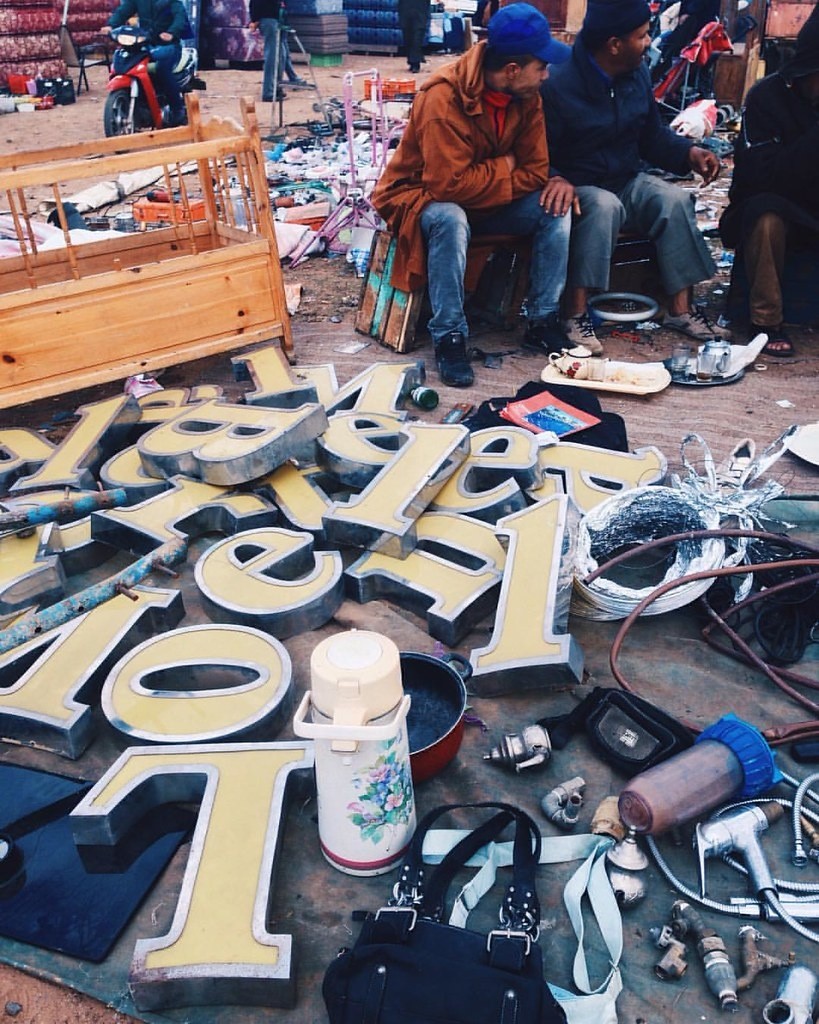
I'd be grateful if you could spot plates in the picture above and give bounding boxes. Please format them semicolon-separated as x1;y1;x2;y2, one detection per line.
784;424;819;465
541;358;671;395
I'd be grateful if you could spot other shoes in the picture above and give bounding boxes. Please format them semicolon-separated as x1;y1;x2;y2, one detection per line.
170;98;185;122
409;64;421;73
263;93;287;102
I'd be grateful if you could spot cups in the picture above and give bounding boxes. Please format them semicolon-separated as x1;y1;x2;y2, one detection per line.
697;353;716;382
587;359;606;381
671;348;690;371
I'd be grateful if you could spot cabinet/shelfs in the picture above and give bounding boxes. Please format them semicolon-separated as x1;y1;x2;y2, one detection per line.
715;0;767;109
472;0;588;46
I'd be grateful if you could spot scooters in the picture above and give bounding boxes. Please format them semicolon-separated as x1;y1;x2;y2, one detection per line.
95;24;208;154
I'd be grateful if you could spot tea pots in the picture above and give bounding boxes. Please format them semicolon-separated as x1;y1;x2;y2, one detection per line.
549;346;592;378
702;336;731;372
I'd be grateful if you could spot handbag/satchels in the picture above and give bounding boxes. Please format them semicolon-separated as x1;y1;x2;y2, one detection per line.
422;829;623;1024
322;800;566;1024
538;686;693;779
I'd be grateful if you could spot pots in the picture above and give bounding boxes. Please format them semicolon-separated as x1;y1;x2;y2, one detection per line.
399;651;473;786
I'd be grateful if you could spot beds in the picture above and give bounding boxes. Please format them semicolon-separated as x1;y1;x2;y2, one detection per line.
0;90;297;415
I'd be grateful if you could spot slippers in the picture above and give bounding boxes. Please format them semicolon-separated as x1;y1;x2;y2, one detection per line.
749;327;795;358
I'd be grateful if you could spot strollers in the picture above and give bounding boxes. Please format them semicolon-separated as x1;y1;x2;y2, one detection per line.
642;0;759;136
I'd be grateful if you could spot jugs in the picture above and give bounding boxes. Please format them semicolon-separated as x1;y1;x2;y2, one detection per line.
290;630;417;877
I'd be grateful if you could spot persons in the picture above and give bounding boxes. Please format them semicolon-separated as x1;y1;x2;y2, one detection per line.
98;0;194;129
370;0;570;390
398;0;430;74
537;2;720;352
718;0;819;357
248;0;308;102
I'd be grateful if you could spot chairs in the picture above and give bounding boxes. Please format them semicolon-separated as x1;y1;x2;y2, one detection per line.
57;24;112;92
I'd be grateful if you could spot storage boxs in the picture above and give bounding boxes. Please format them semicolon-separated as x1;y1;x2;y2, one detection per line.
9;74;35;93
354;228;532;355
587;233;693;327
132;197;206;224
36;78;75;105
364;79;416;101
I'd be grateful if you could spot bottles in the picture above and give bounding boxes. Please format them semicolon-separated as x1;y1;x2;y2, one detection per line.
411;387;438;410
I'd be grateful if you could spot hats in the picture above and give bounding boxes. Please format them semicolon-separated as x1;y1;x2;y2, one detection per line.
487;2;572;63
580;0;651;50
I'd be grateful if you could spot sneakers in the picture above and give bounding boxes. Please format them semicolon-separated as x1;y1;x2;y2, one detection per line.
661;304;732;343
556;310;603;356
434;332;473;386
520;312;577;359
